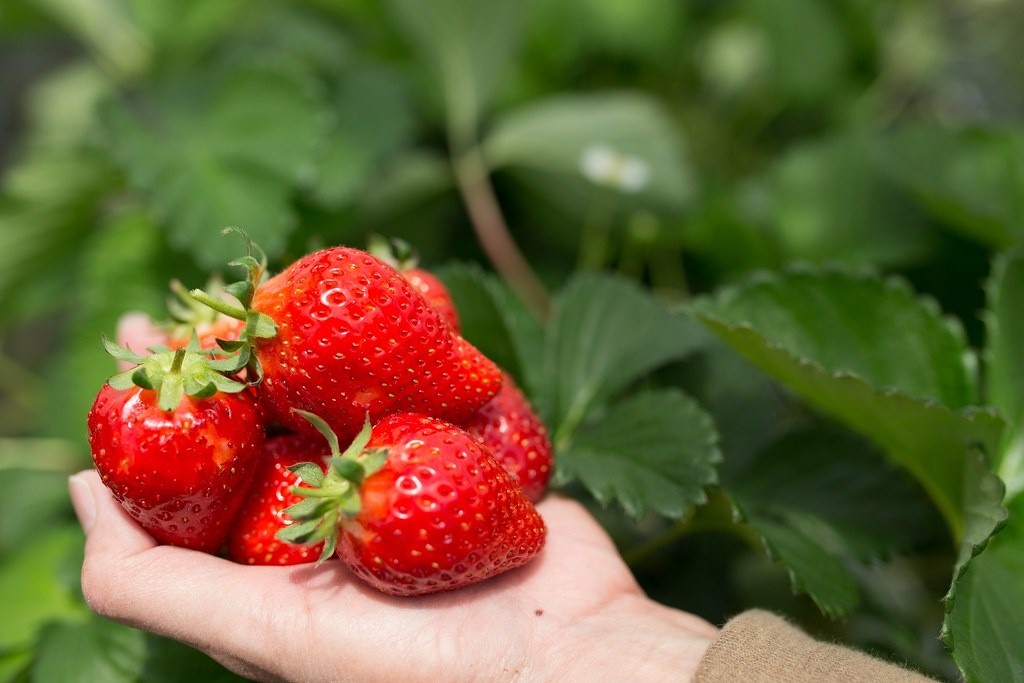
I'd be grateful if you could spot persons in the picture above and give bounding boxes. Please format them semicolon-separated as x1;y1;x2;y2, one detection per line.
67;304;946;683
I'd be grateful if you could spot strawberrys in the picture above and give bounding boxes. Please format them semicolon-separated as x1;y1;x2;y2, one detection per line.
87;236;554;596
